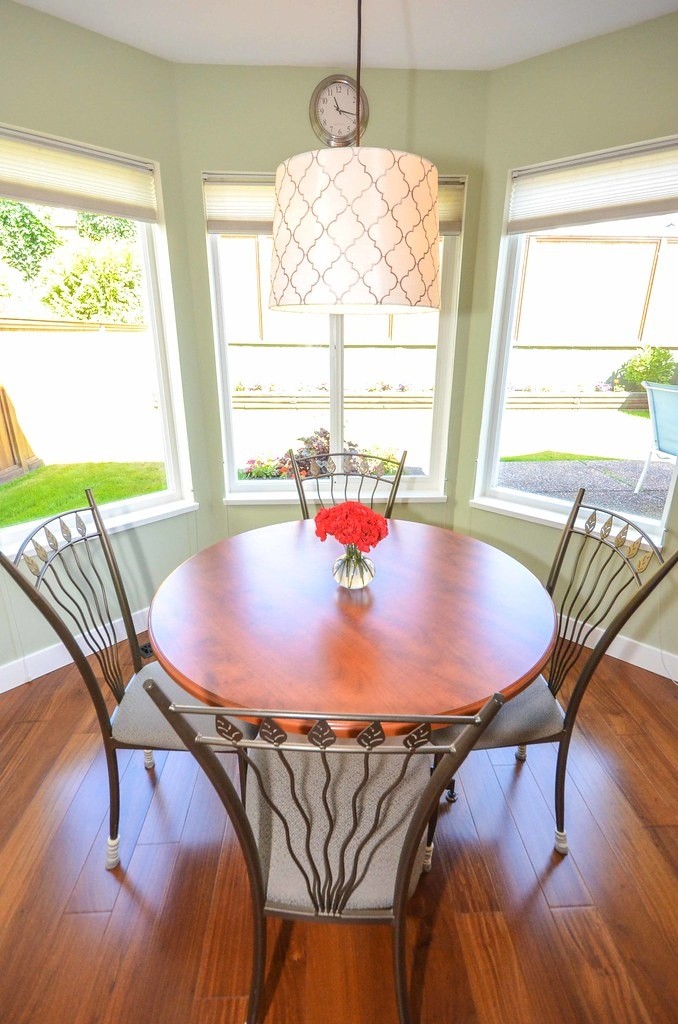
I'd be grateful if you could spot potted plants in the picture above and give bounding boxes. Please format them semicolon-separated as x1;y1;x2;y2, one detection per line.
617;344;678;410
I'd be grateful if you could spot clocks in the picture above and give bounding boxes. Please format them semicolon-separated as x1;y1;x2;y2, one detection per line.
309;73;370;148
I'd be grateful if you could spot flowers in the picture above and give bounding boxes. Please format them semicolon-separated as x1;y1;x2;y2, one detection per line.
314;500;389;553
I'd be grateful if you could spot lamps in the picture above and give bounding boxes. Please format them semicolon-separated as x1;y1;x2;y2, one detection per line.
259;0;441;318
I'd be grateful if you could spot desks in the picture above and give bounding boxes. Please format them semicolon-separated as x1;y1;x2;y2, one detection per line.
146;518;559;741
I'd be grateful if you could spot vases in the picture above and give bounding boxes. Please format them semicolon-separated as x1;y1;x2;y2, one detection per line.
332;543;373;590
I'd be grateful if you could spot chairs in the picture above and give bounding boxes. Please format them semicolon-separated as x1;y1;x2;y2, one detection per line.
407;487;678;872
142;678;507;1024
287;447;408;521
632;380;678;493
1;488;261;868
